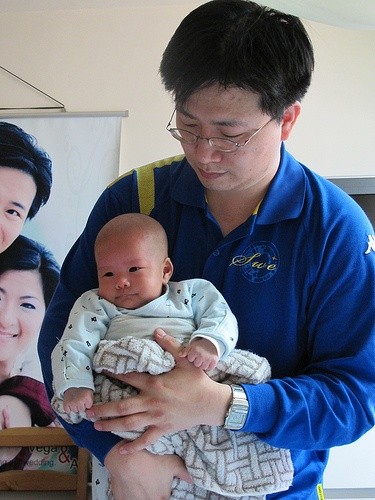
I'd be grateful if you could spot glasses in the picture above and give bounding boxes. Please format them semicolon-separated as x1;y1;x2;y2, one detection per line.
165;106;276;153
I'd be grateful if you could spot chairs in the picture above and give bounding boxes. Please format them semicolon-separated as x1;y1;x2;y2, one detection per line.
0;426;88;500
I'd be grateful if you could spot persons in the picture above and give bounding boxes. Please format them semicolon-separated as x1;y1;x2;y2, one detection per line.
51;213;295;499
36;0;375;500
0;122;60;470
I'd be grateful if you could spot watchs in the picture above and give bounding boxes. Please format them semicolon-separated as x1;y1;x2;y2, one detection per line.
224;383;249;430
